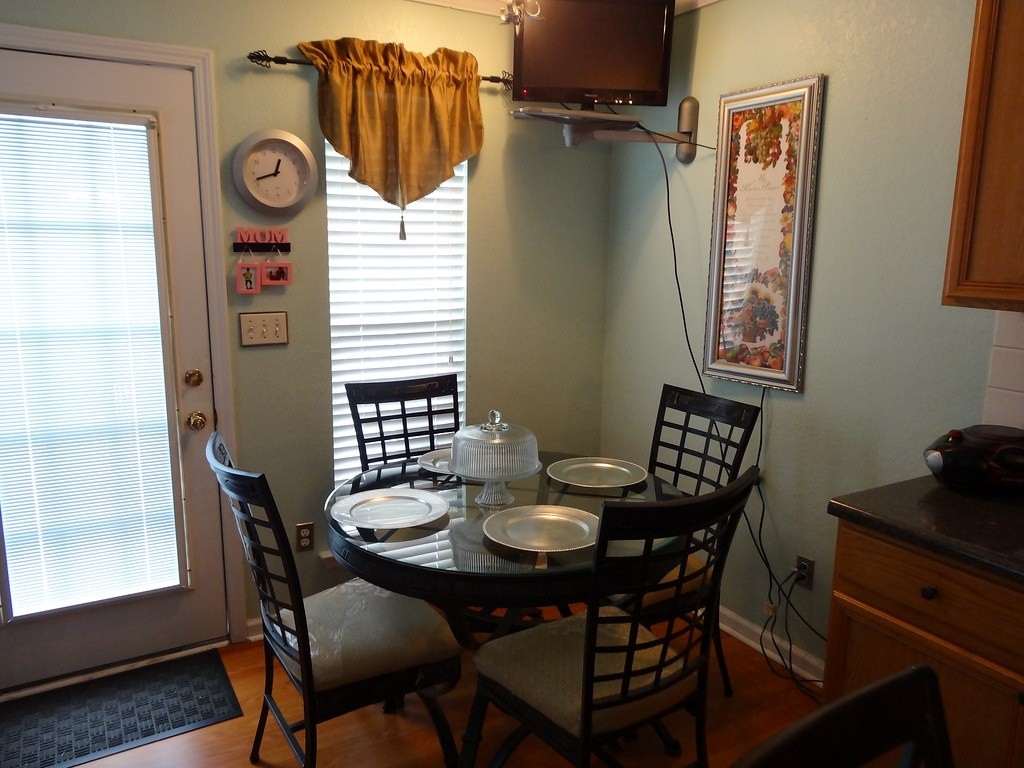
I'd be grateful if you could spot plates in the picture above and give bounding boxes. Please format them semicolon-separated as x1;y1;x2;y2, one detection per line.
545;455;649;487
418;447;455;474
330;488;450;529
482;504;603;552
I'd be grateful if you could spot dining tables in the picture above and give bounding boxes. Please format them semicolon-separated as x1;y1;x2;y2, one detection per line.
323;452;688;768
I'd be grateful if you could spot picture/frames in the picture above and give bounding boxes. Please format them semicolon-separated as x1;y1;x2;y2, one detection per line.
701;73;824;394
236;263;261;294
261;262;292;286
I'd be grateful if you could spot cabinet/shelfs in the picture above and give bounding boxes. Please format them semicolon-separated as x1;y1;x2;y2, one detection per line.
820;519;1024;768
939;0;1024;313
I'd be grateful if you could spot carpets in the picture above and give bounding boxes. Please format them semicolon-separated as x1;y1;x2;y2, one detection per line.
0;648;243;768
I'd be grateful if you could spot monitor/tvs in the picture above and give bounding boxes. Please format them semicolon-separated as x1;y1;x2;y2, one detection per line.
511;1;676;111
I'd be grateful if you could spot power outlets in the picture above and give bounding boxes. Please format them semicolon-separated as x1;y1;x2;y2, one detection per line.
296;521;314;552
795;554;814;590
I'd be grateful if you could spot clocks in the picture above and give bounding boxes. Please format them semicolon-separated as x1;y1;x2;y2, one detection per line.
233;128;318;216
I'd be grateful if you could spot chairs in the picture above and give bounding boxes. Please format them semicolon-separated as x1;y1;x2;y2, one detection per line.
345;374;459;473
606;384;760;699
460;464;760;768
205;430;463;768
726;663;955;768
